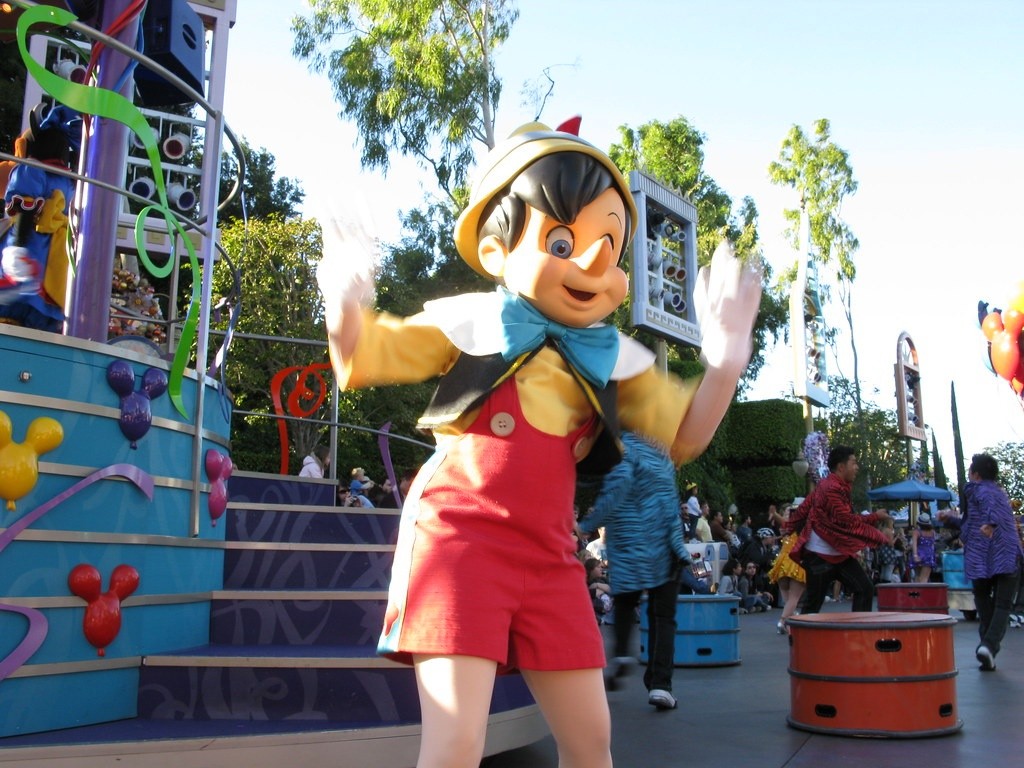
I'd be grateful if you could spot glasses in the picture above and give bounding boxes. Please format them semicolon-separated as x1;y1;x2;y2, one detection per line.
748;566;756;569
681;507;687;510
339;489;351;494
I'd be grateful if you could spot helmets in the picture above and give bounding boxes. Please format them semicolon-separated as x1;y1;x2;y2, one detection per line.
757;528;774;539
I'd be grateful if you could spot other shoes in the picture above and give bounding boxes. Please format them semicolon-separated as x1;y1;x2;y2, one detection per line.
739;592;844;613
1009;621;1021;628
776;622;788;635
602;656;638;683
977;646;996;671
648;689;677;709
1018;616;1024;623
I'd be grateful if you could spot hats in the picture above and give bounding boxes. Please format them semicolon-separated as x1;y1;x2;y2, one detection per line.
916;513;931;525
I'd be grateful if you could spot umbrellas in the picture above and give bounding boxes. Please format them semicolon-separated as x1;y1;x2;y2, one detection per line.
868;479;950;513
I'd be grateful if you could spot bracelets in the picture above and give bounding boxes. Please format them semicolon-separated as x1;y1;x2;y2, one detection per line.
773;513;778;518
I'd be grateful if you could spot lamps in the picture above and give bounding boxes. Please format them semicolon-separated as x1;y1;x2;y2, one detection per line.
52;44;85;84
128;113;196;211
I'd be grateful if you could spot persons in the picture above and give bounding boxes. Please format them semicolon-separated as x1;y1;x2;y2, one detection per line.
936;454;1024;670
678;479;792;616
335;466;418;509
577;433;693;709
318;115;763;768
298;445;330;478
861;505;937;582
572;505;612;625
769;445;892;614
40;105;89;162
765;496;806;634
